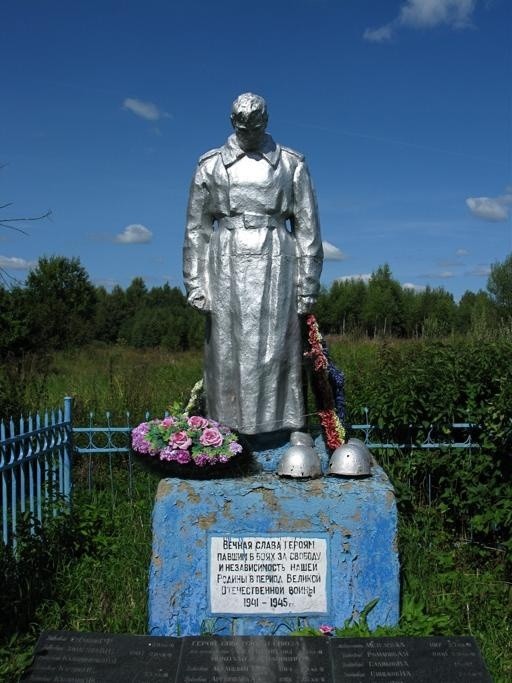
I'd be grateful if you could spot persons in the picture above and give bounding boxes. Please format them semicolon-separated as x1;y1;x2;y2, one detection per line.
182;89;323;439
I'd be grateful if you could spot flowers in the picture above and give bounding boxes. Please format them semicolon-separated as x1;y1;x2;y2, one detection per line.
130;313;352;467
